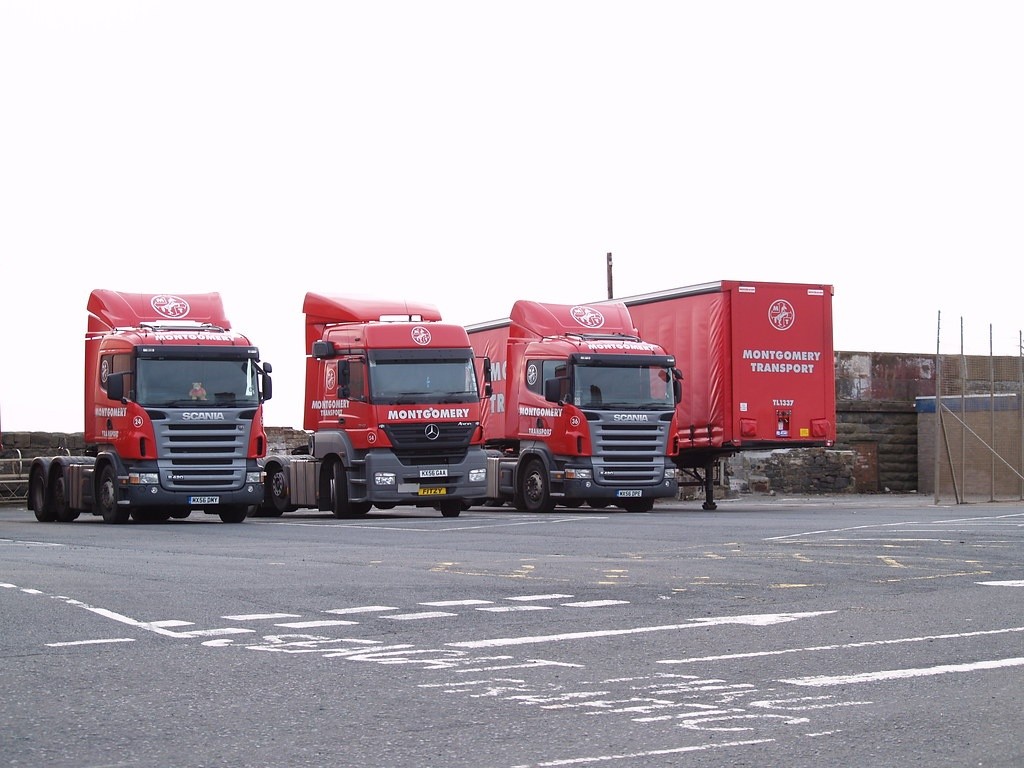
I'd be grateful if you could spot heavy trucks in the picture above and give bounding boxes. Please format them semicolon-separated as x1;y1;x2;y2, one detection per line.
458;299;683;514
26;290;273;526
247;291;489;520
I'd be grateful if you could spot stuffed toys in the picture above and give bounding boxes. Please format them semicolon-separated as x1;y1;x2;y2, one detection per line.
189;382;207;400
